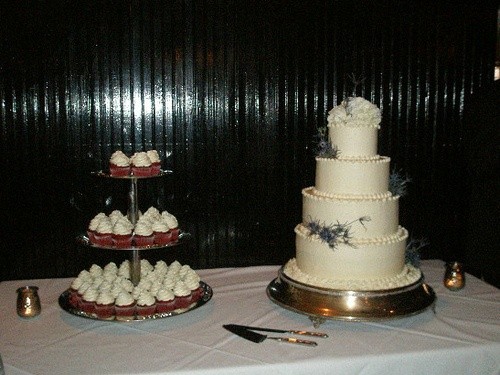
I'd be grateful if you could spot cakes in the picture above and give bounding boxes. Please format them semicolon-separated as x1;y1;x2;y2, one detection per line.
282;97;423;292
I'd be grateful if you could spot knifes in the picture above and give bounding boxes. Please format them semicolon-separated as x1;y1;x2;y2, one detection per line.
241;325;329;339
222;325;318;348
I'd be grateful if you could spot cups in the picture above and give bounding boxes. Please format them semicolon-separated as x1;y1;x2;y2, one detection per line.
16;286;41;318
444;261;465;292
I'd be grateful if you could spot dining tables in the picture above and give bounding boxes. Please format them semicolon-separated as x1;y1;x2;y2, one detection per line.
0;260;500;375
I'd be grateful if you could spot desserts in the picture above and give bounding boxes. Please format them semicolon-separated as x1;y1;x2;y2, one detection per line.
86;206;180;248
108;150;163;178
69;259;204;316
